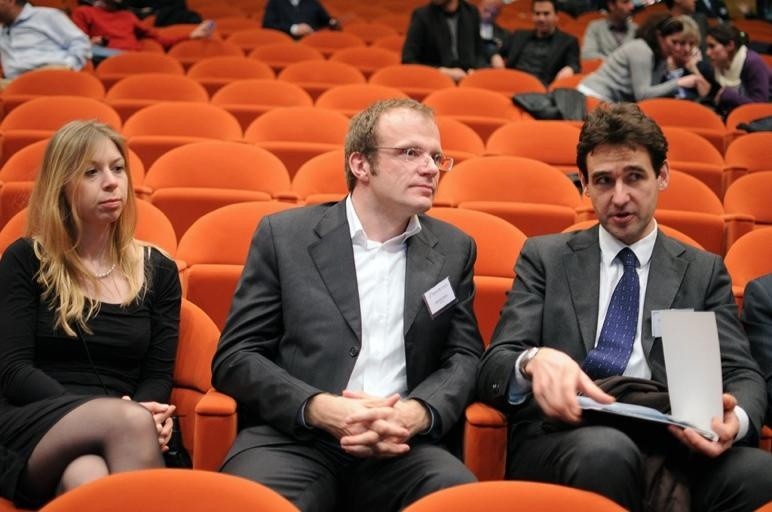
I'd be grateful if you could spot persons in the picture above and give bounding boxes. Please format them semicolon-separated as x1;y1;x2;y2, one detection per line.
261;1;331;41
209;96;487;511
741;273;772;430
478;97;771;511
0;117;195;512
0;0;216;80
402;0;772;120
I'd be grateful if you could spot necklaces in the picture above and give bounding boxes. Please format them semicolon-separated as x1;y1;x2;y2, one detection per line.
94;264;115;279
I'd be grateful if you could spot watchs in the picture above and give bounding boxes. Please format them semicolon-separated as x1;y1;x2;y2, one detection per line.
518;346;539;380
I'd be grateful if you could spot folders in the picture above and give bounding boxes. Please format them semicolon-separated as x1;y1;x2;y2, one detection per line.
575;309;726;449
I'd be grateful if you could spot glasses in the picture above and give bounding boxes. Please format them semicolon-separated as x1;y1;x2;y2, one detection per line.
362;146;454;172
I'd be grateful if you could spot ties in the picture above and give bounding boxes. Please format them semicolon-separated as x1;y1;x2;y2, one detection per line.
579;247;641;382
